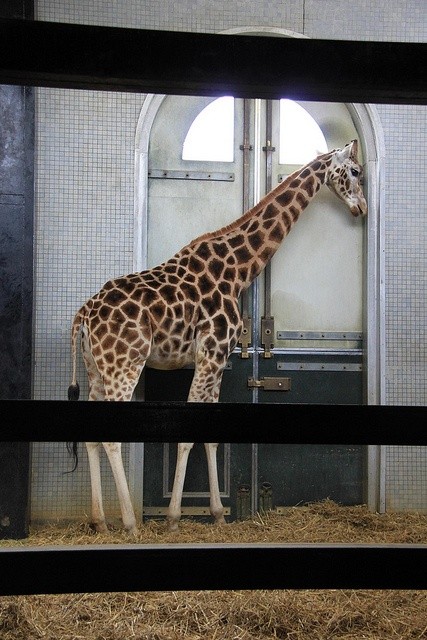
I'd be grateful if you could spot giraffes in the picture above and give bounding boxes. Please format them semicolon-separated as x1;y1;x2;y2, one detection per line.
62;137;369;539
